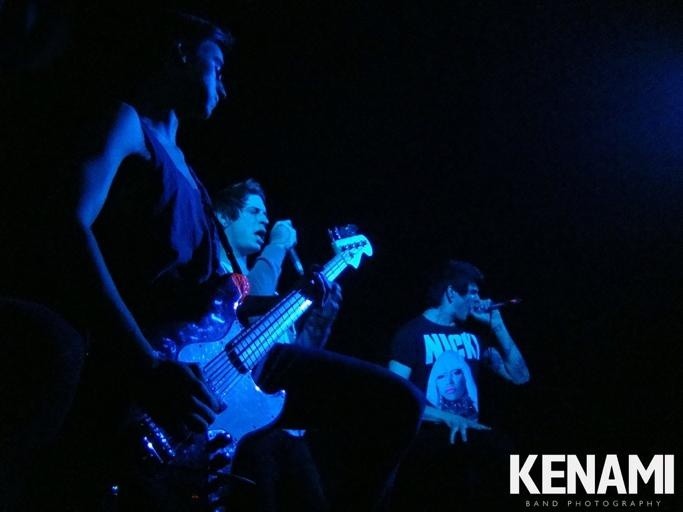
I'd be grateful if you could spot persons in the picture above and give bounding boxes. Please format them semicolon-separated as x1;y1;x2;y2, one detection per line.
211;175;343;512
380;259;533;512
0;298;89;509
423;350;483;421
64;11;334;509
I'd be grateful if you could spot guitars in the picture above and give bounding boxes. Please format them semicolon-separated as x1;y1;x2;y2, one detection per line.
112;223;373;512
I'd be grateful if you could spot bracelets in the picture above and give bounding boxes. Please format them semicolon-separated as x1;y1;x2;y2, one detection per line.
138;348;163;385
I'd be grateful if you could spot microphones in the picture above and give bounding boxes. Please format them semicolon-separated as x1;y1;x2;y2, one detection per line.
474;298;521;313
288;246;307;277
